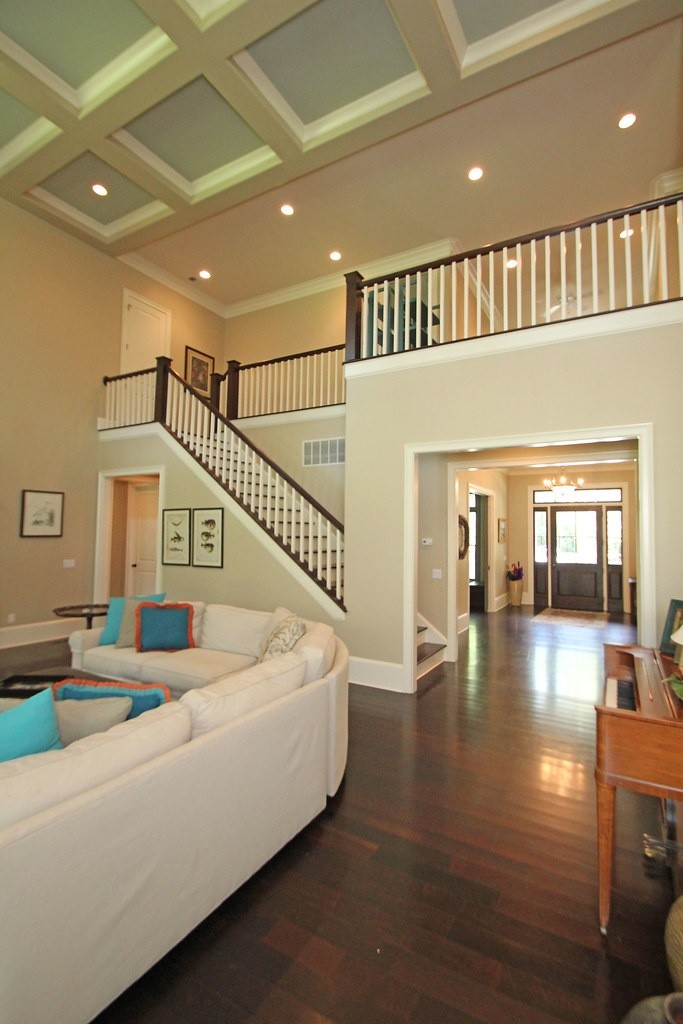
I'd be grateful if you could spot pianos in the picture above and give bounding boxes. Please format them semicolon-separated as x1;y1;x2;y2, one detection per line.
593;641;683;934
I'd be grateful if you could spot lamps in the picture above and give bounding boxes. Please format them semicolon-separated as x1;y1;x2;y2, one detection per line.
543;466;583;496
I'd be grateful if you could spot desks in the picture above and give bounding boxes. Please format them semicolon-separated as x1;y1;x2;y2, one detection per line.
52;604;108;629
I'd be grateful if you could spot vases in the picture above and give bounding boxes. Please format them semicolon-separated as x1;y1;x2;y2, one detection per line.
509;579;522;606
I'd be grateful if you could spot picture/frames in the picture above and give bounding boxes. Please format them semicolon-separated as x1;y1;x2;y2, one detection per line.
183;345;215;400
192;508;224;568
660;599;683;653
20;489;64;537
162;508;191;566
498;518;507;544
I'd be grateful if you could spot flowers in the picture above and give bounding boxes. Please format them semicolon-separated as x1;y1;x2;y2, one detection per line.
505;561;524;580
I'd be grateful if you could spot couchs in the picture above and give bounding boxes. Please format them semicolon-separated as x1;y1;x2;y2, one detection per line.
0;603;349;1024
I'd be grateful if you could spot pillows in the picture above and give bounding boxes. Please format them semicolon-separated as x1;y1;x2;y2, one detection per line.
54;696;133;748
257;606;305;664
115;598;177;648
95;593;165;646
0;686;65;763
166;601;205;647
52;678;171;721
134;602;194;653
